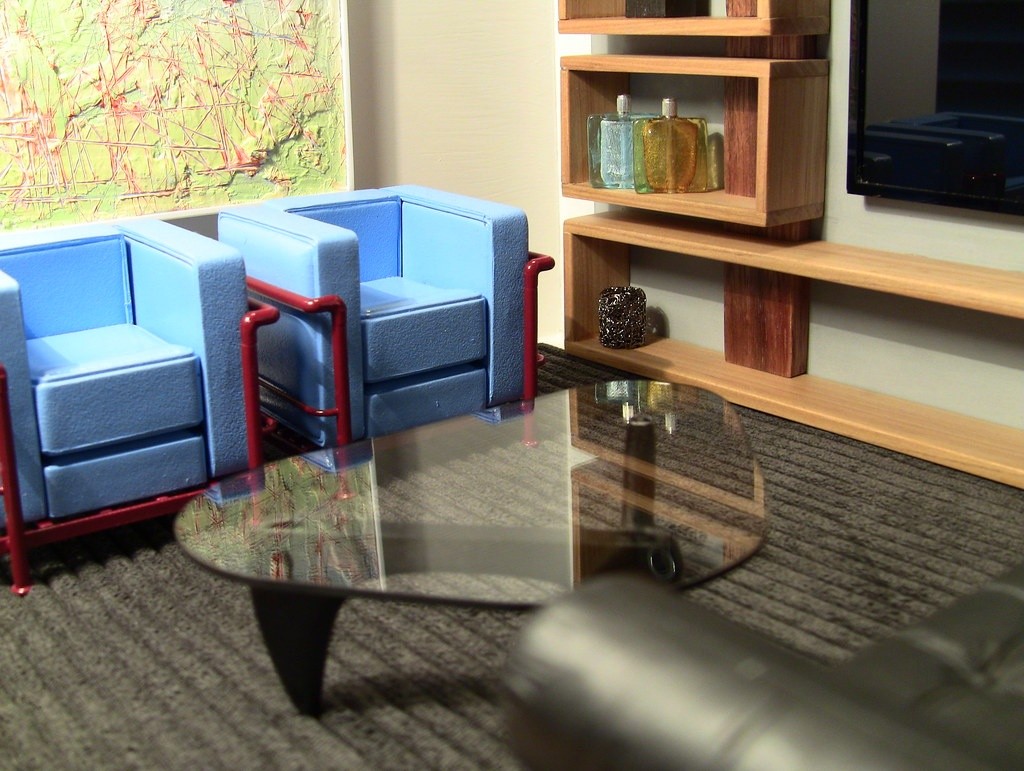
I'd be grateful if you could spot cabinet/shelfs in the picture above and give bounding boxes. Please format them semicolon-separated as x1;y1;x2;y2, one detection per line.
559;0;1024;491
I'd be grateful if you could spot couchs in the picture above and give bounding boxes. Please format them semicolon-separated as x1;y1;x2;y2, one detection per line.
0;213;278;600
502;554;1024;771
218;182;554;452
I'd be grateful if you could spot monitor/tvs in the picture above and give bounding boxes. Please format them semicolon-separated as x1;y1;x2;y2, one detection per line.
847;0;1024;215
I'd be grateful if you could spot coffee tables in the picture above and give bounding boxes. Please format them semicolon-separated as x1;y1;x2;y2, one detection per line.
171;379;769;718
